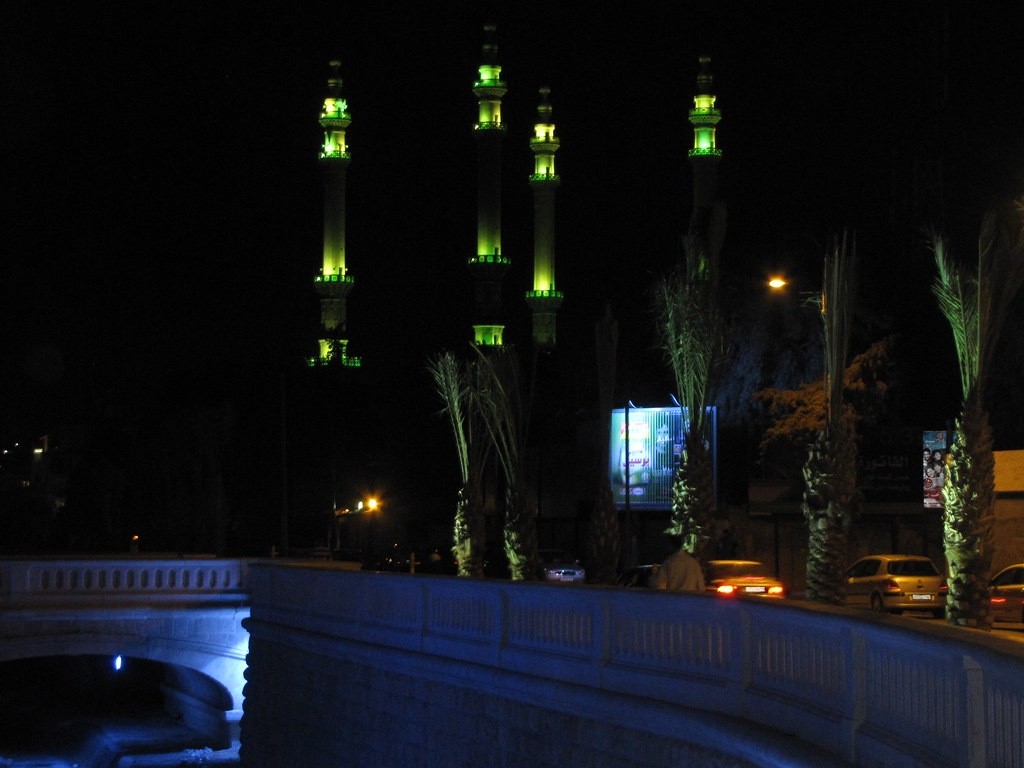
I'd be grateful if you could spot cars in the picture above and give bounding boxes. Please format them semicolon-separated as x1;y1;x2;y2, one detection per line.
613;563;663;590
991;563;1023;627
701;559;785;597
846;553;949;616
535;548;586;584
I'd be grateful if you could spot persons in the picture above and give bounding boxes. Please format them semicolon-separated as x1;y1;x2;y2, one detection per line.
419;554;449;575
653;537;693;591
924;448;945;492
